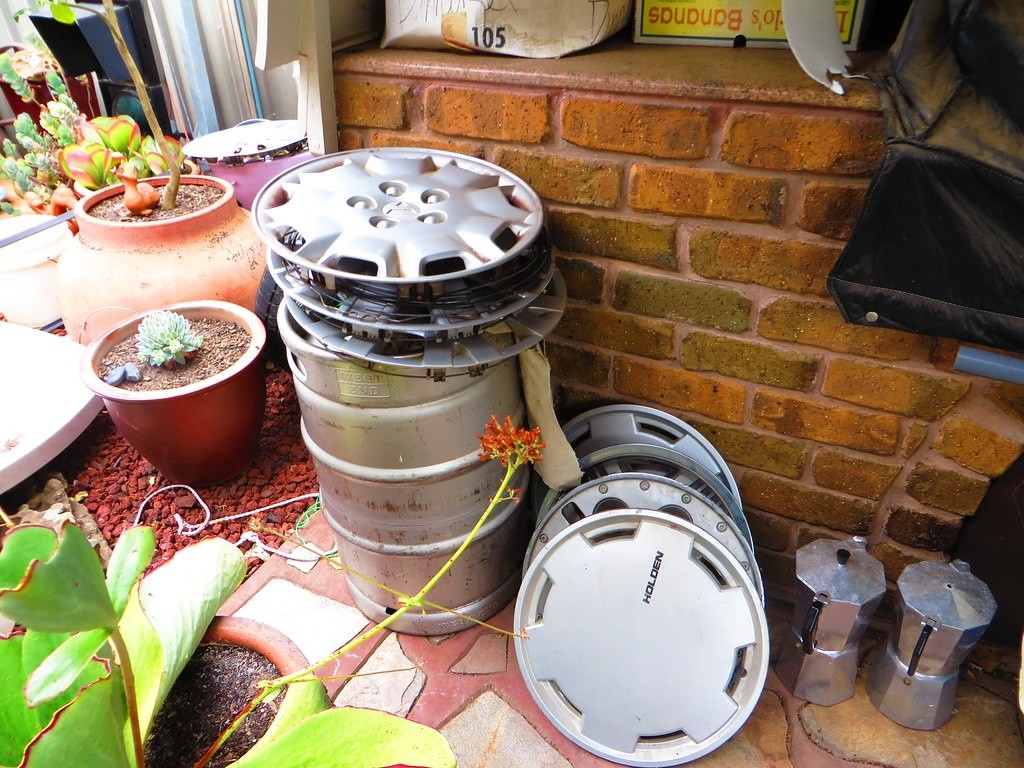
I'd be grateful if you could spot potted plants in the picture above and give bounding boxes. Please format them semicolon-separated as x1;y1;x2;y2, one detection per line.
0;0;269;350
0;416;546;768
83;299;268;482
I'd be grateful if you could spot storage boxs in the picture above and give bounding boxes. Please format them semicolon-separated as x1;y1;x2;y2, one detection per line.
632;0;865;51
380;0;634;60
